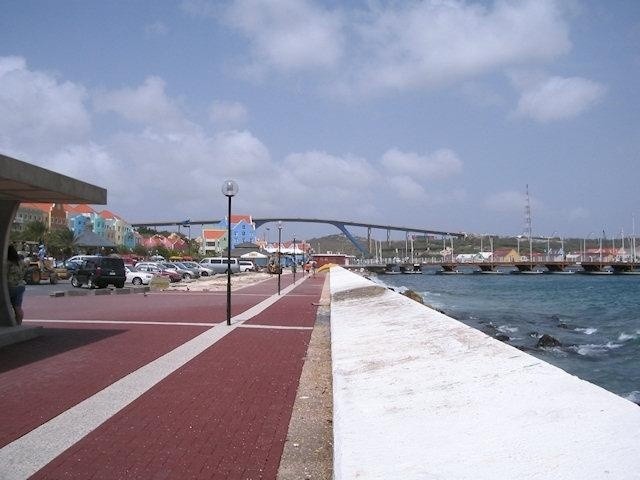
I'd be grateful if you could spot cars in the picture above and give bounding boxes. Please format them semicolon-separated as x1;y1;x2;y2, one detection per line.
124;255;256;286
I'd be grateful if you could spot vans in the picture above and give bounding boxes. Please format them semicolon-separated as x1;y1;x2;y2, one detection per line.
68;254;100;265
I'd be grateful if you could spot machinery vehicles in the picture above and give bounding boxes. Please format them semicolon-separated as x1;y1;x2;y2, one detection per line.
14;240;71;285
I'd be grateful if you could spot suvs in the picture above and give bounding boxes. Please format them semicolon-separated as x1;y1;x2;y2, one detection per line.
70;256;127;289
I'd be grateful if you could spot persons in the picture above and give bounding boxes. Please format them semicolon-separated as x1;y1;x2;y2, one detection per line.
311;259;318;280
8;244;26;326
305;261;310;279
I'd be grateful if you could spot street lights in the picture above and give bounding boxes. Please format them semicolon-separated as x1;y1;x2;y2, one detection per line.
291;231;298;286
276;220;286;295
221;179;239;326
